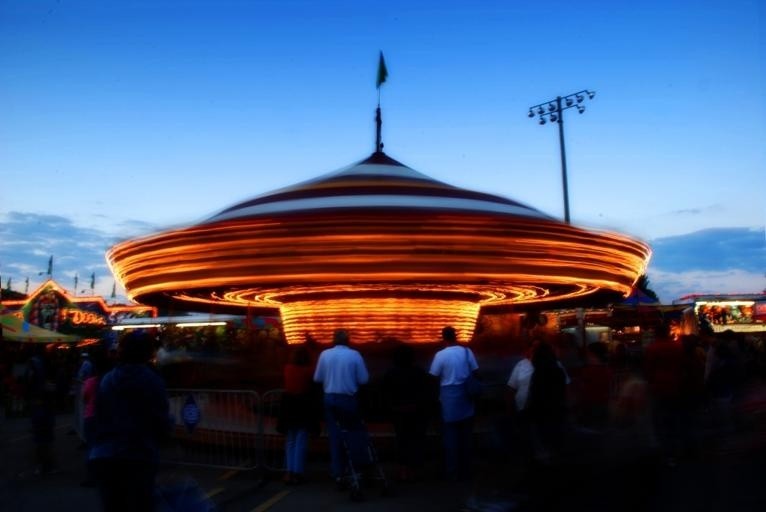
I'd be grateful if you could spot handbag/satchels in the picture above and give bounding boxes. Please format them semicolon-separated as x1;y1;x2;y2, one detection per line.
463;374;484;399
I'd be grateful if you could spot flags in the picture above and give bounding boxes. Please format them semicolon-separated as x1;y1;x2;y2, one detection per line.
376;52;390;88
